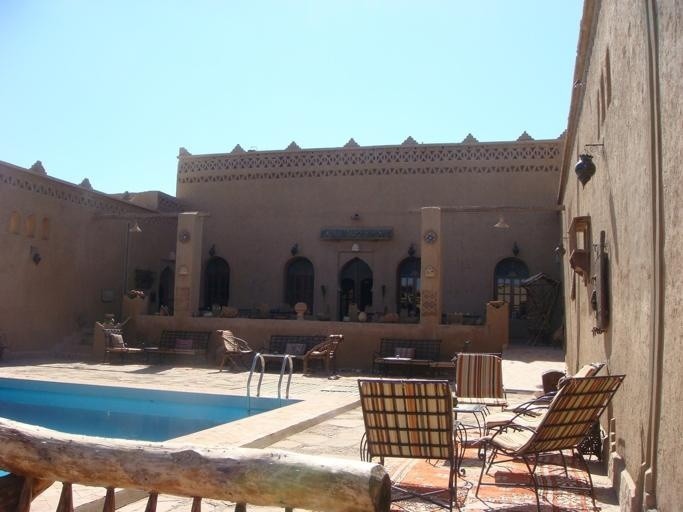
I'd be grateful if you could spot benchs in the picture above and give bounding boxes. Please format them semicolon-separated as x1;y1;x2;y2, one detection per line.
261;333;329;373
143;329;212;369
370;337;442;378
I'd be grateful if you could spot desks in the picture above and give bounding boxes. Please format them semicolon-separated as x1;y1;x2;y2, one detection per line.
452;397;490;462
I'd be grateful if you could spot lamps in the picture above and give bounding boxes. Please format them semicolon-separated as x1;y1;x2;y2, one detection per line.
206;243;216;256
574;143;605;190
128;221;142;233
511;240;519;255
289;241;299;257
350;243;360;252
492;215;510;229
407;243;417;256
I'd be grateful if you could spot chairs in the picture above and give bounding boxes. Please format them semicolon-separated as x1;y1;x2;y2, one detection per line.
102;326;143;365
454;353;510;412
357;377;468;512
302;334;345;373
471;361;628;512
214;328;254;373
